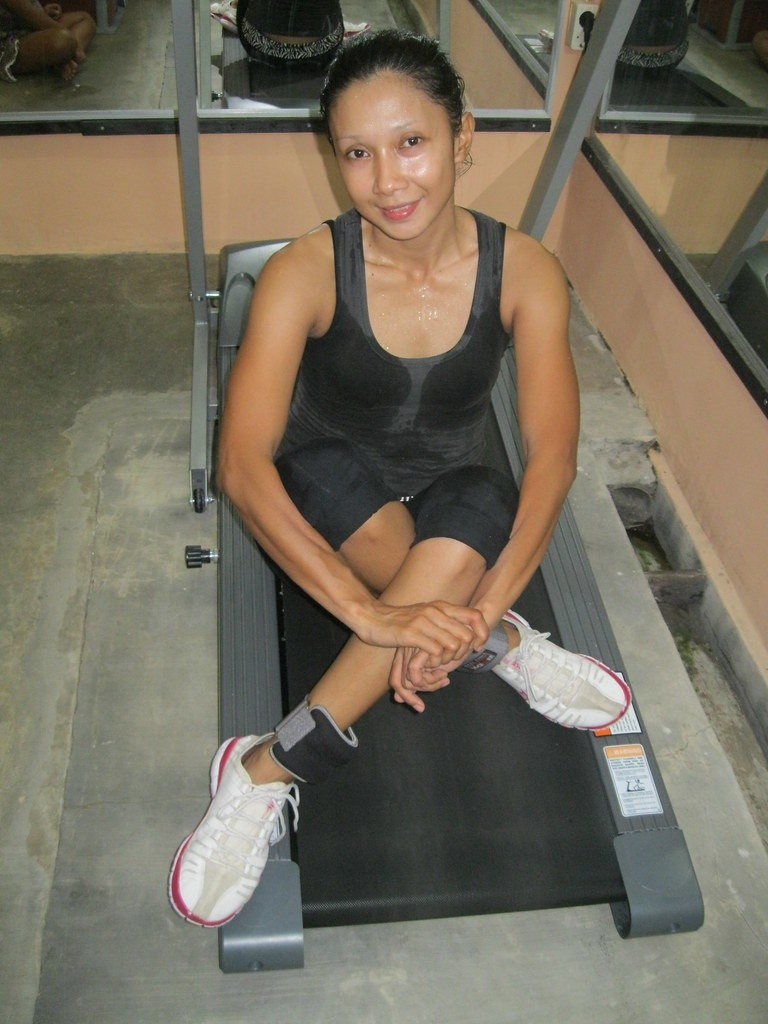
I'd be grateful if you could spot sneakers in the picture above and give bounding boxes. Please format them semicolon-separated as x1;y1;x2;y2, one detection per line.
491;609;631;732
538;29;554;48
343;21;371;41
168;733;300;928
210;0;238;34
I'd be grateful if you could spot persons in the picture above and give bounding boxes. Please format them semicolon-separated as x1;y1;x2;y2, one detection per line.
168;27;632;927
0;0;98;81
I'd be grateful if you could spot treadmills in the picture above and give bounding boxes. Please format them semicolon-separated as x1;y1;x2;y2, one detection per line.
197;0;450;110
609;54;730;111
165;0;710;975
